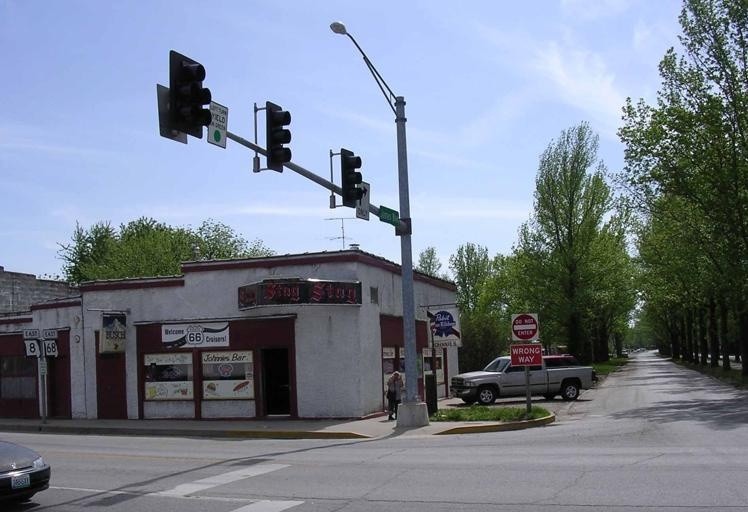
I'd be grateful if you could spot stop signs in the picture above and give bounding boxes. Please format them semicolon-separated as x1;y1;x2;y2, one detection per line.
510;314;538;341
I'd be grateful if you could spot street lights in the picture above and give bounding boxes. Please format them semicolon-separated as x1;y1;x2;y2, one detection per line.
329;21;420;399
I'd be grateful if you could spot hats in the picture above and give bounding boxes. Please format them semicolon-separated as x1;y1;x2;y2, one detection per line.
392;371;400;379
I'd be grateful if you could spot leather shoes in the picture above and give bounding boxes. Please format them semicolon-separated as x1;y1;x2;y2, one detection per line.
389;417;394;420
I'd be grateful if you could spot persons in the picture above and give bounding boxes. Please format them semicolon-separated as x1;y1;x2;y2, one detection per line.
386;370;405;421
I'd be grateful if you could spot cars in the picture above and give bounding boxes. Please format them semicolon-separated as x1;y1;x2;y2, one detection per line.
1;438;50;508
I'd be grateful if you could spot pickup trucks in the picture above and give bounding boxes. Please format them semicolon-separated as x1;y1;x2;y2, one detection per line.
450;356;598;401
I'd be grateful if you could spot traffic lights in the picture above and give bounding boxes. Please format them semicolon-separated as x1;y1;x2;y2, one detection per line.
264;101;291;174
340;149;362;209
168;51;212;139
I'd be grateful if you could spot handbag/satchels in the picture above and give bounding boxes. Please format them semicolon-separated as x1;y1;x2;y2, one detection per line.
387;390;396;402
